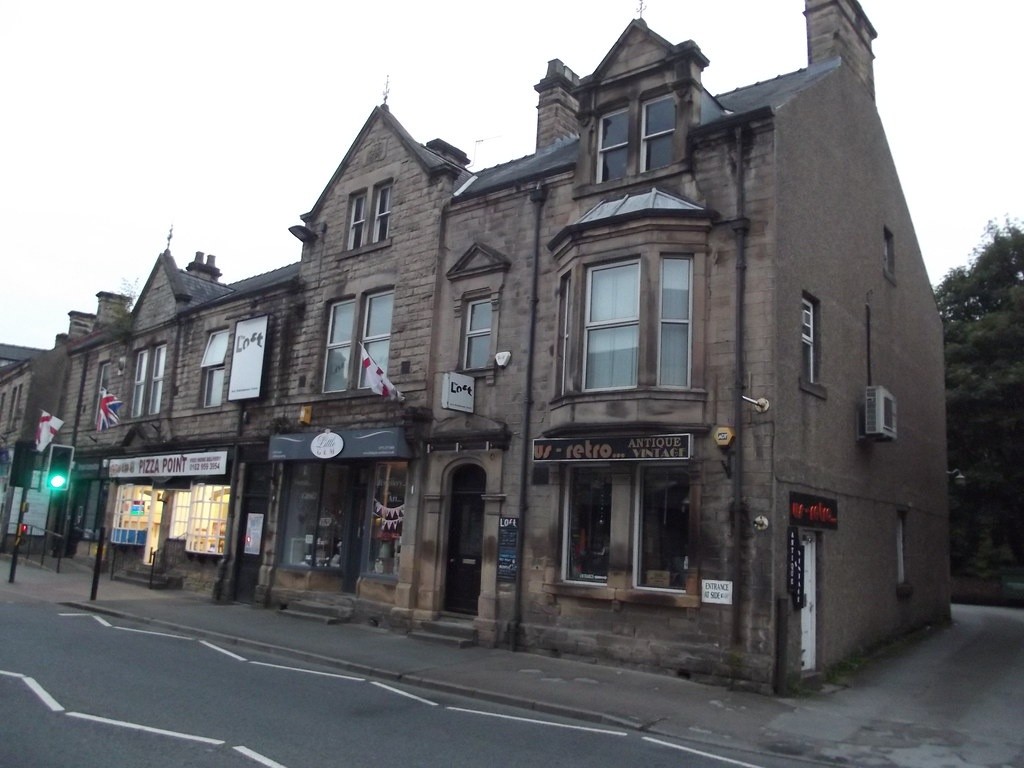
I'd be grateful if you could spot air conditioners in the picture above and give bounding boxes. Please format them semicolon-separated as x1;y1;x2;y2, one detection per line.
866;385;898;440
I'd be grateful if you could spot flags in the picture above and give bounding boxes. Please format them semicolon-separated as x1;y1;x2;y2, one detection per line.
361;342;405;401
35;409;65;454
96;388;124;432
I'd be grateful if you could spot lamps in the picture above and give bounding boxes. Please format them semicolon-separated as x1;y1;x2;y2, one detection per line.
945;469;965;487
288;225;318;243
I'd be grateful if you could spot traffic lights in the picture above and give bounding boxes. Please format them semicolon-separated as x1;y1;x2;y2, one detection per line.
44;444;74;489
